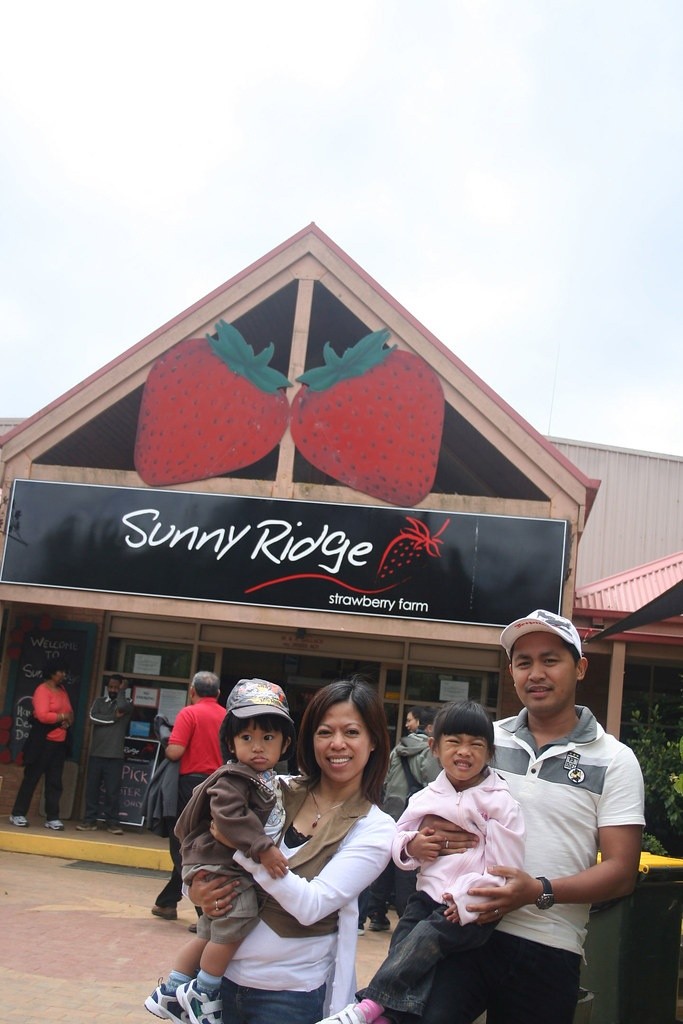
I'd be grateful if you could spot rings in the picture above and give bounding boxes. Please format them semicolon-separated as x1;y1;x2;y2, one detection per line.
445;840;449;849
495;909;501;918
215;901;220;912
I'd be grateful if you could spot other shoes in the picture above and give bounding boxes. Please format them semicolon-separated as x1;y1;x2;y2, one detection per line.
315;1004;366;1024
370;917;390;930
358;924;364;935
76;822;97;831
106;825;124;834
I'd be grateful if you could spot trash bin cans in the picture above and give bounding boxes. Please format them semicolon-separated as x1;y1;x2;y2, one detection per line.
575;850;683;1024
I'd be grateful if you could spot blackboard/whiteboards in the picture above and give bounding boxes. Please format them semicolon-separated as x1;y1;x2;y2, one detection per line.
95;736;162;827
8;627;88;760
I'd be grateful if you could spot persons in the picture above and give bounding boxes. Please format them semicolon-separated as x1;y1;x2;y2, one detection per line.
9;659;74;830
75;674;134;834
317;700;524;1024
143;679;297;1024
419;610;641;1024
352;706;442;934
152;671;226;934
188;680;396;1024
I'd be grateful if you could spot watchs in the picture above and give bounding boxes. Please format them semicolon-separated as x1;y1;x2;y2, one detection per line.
536;877;555;909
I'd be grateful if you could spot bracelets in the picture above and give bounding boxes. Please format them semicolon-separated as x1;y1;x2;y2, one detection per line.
61;712;65;720
404;844;414;859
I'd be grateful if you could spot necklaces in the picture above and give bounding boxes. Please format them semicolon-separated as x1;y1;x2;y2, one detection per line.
308;788;348;828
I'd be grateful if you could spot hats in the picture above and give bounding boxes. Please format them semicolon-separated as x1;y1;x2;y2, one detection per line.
227;678;294;723
500;609;581;661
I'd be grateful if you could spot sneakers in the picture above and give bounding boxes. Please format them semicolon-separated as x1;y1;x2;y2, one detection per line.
144;977;190;1024
152;906;177;920
9;815;28;826
188;923;197;933
176;978;223;1024
44;820;64;830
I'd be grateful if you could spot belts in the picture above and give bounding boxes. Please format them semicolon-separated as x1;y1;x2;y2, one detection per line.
189;772;210;779
